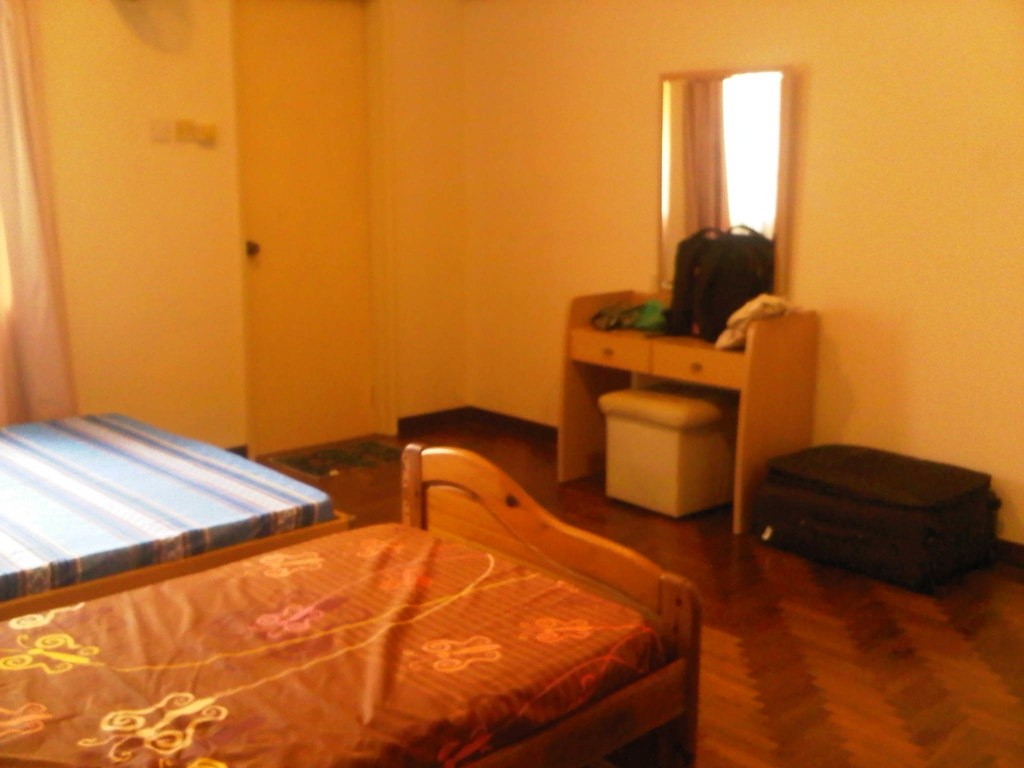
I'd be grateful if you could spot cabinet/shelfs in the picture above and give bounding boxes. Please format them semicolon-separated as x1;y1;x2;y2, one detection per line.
553;283;818;544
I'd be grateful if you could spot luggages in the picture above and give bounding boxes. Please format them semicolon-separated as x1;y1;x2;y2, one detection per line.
754;444;1003;591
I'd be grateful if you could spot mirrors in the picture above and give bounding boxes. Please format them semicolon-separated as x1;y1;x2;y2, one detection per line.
657;67;796;332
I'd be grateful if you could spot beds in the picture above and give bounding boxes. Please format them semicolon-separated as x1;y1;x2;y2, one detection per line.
3;403;355;615
0;429;706;766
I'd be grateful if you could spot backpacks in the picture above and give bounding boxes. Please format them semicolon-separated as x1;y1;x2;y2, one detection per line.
671;227;720;333
691;225;775;343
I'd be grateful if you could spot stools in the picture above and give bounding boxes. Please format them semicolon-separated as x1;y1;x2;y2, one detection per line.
593;388;736;522
